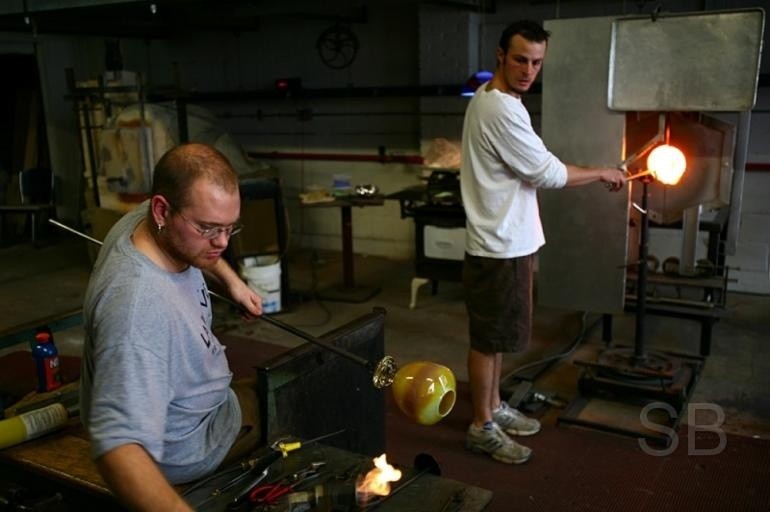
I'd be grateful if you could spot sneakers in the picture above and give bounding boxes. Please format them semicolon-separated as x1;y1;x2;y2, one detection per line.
467;423;533;466
490;402;542;436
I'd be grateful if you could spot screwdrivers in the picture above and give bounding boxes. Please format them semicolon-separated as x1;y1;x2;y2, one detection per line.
270;424;350;459
265;472;321;502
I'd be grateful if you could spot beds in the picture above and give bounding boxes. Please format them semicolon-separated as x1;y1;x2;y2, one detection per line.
299;190;385;304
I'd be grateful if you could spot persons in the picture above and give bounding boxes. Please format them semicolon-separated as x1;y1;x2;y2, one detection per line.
81;142;261;512
461;26;628;465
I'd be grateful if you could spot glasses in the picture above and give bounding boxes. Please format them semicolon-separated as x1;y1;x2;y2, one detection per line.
175;209;244;241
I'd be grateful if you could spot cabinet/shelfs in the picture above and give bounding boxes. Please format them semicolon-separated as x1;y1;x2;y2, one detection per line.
383;180;466;310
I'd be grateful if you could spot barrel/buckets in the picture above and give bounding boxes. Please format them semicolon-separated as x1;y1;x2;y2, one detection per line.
236;254;285;315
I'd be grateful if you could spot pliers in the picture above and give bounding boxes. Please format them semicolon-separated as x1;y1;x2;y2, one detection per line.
249;466;314;505
